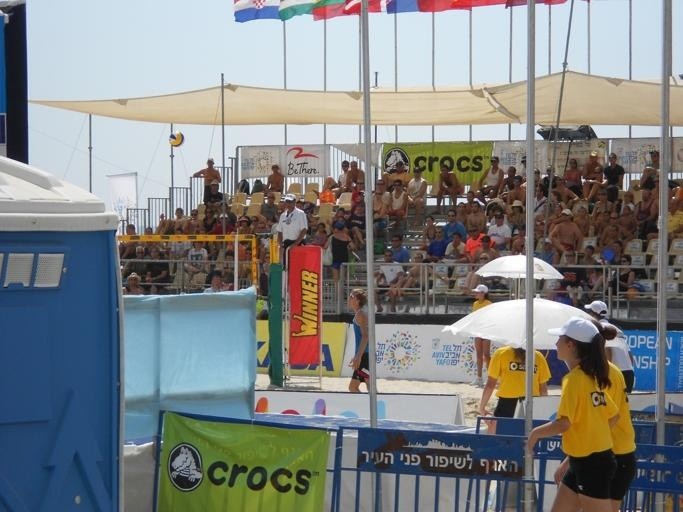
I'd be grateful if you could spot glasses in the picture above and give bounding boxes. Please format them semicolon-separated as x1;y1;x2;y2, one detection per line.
191;213;196;216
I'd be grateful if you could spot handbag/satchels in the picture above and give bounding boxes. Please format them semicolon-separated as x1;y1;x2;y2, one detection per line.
323;248;333;266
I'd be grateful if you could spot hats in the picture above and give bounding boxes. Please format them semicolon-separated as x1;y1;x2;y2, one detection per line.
238;217;248;221
493;211;504;216
220;214;228;218
561;209;572;216
547;318;599;343
333;222;344;229
491;156;498;162
540;238;551;244
284;194;296;201
127;273;141;282
509;200;525;214
584;301;608;317
471;284;488;294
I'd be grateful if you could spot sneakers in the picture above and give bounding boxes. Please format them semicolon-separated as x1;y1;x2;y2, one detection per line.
470;376;488;385
567;285;582;300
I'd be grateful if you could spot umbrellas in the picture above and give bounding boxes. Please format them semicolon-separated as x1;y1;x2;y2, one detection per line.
441;295;598;350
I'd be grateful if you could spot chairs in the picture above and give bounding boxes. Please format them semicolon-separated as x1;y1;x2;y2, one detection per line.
431;261;474;296
196;183;352;236
534;179;683;299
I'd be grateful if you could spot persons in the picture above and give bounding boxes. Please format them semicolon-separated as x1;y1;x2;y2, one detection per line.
479;345;552;435
584;300;634;393
348;288;378;393
527;315;621;512
554;318;637;512
469;285;494;385
119;151;683;312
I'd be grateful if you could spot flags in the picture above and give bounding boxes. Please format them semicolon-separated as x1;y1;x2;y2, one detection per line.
233;0;567;23
